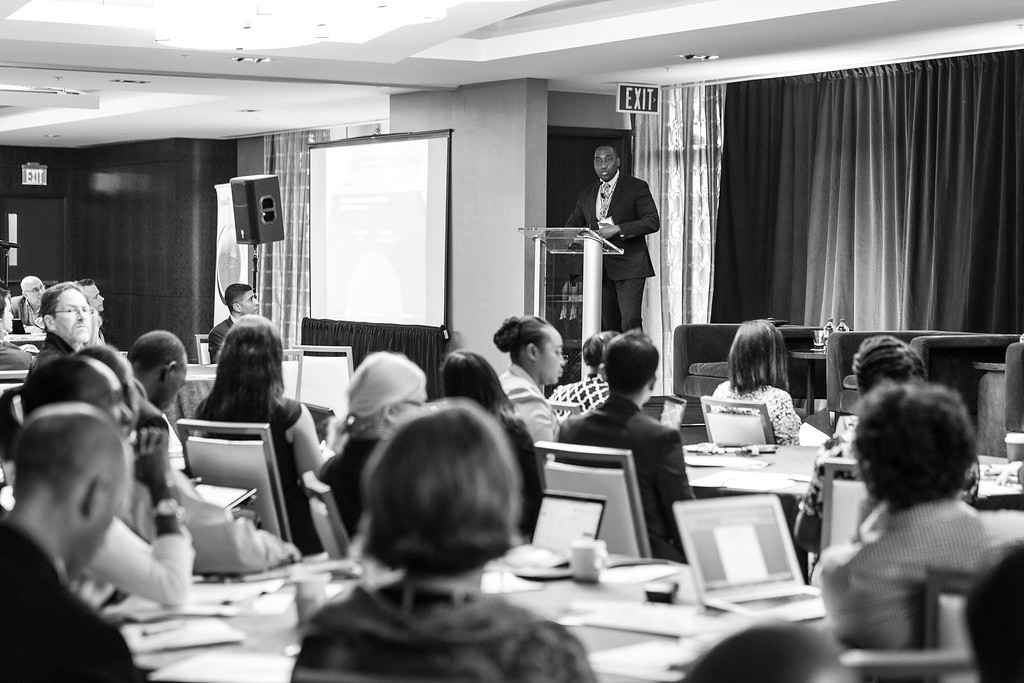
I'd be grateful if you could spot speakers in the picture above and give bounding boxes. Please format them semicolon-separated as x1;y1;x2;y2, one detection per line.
230;175;284;246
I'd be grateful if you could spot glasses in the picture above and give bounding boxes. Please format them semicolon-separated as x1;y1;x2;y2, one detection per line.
24;285;46;293
48;307;94;317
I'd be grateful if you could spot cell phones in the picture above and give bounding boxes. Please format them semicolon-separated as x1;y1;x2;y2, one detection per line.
645;582;679;603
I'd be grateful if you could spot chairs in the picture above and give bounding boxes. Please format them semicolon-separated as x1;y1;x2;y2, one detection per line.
0;332;977;683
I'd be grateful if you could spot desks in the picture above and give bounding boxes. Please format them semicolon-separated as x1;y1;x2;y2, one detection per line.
684;442;1023;583
119;557;826;683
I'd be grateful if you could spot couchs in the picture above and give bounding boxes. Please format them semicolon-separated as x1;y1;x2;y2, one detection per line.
673;320;1024;459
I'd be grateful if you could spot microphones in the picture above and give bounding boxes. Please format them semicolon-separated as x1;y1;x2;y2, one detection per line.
601;193;605;198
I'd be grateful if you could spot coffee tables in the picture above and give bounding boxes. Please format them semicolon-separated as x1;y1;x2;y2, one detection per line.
790;350;826;418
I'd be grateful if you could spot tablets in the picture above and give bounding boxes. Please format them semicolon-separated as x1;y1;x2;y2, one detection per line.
194;483;258;511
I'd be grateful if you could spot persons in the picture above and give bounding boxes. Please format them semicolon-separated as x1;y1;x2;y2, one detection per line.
192;313;325;556
555;328;696;561
711;320;800;445
817;334;1024;651
76;279;105;348
0;283;33;370
565;144;660;334
548;330;620;419
31;282;91;377
317;351;428;540
20;346;196;609
208;284;259;365
442;351;543;535
493;315;566;442
10;275;45;325
126;330;188;469
0;404;132;683
289;402;597;683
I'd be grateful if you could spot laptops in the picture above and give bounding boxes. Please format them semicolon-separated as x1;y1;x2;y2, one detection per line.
671;493;826;623
533;489;608;553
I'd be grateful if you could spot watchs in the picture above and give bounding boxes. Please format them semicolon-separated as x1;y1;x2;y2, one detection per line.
152;499;177;518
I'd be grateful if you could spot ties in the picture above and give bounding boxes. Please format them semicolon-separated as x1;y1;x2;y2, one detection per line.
599;183;609;222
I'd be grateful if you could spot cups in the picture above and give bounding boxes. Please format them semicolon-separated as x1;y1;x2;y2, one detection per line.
1005;432;1024;463
294;573;333;622
569;539;608;583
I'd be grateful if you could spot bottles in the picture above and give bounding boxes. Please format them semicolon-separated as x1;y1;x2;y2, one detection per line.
837;319;850;332
824;318;837;352
